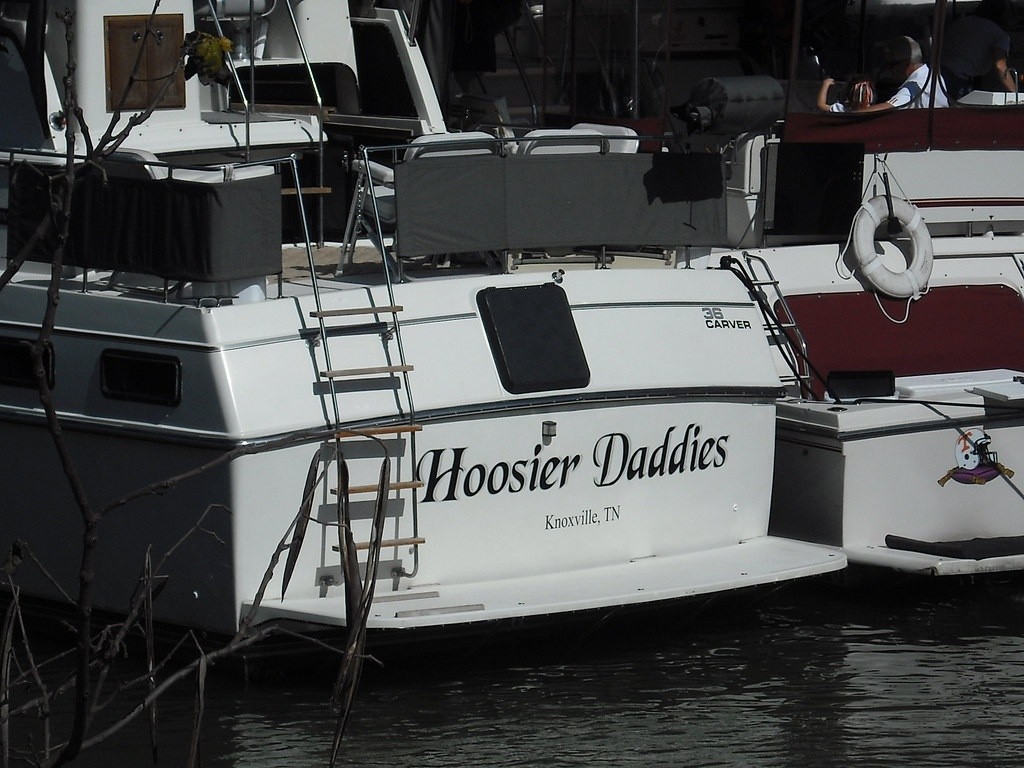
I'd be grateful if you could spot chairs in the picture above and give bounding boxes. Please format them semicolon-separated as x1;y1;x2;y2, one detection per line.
333;121;640;278
0;145;284;300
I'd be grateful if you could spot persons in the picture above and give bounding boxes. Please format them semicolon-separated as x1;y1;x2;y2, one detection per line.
941;0;1015;100
848;36;950;113
816;73;874;113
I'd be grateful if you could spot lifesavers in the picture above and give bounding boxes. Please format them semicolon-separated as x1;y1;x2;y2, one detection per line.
850;193;935;299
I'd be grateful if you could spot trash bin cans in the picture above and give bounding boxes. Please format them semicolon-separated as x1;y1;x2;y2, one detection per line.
173;161;276;303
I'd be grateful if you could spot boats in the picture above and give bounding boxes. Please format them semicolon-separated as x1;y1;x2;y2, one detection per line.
0;0;849;651
461;0;1023;577
